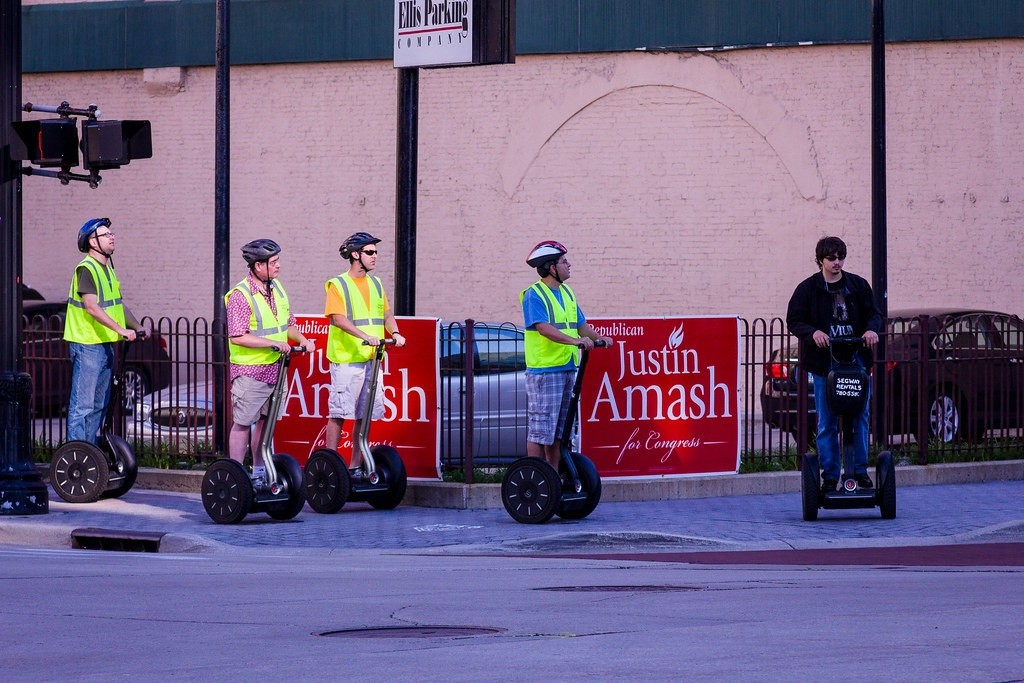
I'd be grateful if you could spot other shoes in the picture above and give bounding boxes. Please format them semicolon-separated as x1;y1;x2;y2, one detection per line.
348;466;365;476
248;473;265;491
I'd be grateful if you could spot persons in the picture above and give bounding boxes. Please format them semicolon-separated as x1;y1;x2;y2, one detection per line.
325;232;406;469
64;218;151;444
785;236;883;492
519;241;613;464
224;239;315;479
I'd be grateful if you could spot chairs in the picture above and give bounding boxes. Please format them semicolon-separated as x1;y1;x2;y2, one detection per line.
953;332;977;348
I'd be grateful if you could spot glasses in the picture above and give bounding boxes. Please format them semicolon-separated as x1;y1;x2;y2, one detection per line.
359;249;377;255
824;253;846;260
91;231;115;238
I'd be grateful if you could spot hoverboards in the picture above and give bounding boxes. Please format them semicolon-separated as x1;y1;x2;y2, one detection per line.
498;340;603;524
202;346;309;526
302;339;400;510
798;338;897;517
50;332;149;503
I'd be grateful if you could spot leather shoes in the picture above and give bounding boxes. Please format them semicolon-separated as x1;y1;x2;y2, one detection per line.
822;478;838;490
853;472;874;488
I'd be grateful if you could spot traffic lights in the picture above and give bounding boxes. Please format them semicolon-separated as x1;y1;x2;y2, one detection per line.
17;117;79;168
82;118;153;170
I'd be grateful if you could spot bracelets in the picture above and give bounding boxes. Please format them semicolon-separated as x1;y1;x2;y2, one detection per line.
392;332;399;335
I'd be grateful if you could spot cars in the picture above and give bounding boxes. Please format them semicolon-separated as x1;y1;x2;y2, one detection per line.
441;323;582;468
22;297;170;418
760;306;1024;447
120;315;576;467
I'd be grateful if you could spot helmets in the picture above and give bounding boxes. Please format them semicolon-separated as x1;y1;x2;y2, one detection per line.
77;216;111;252
526;240;567;267
240;239;281;262
339;232;382;258
